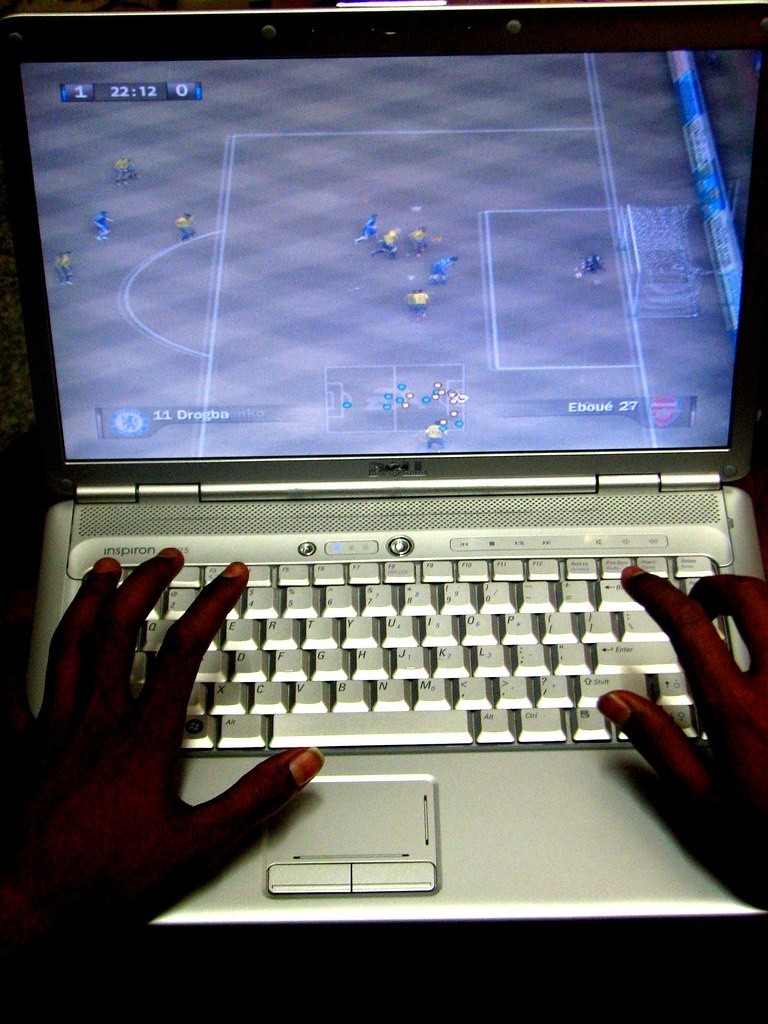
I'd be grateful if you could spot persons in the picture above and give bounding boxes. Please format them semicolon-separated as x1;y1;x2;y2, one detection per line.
426;420;447;451
52;157;607;323
0;549;768;1024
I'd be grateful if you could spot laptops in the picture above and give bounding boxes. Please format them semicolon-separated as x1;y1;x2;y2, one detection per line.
0;4;768;926
